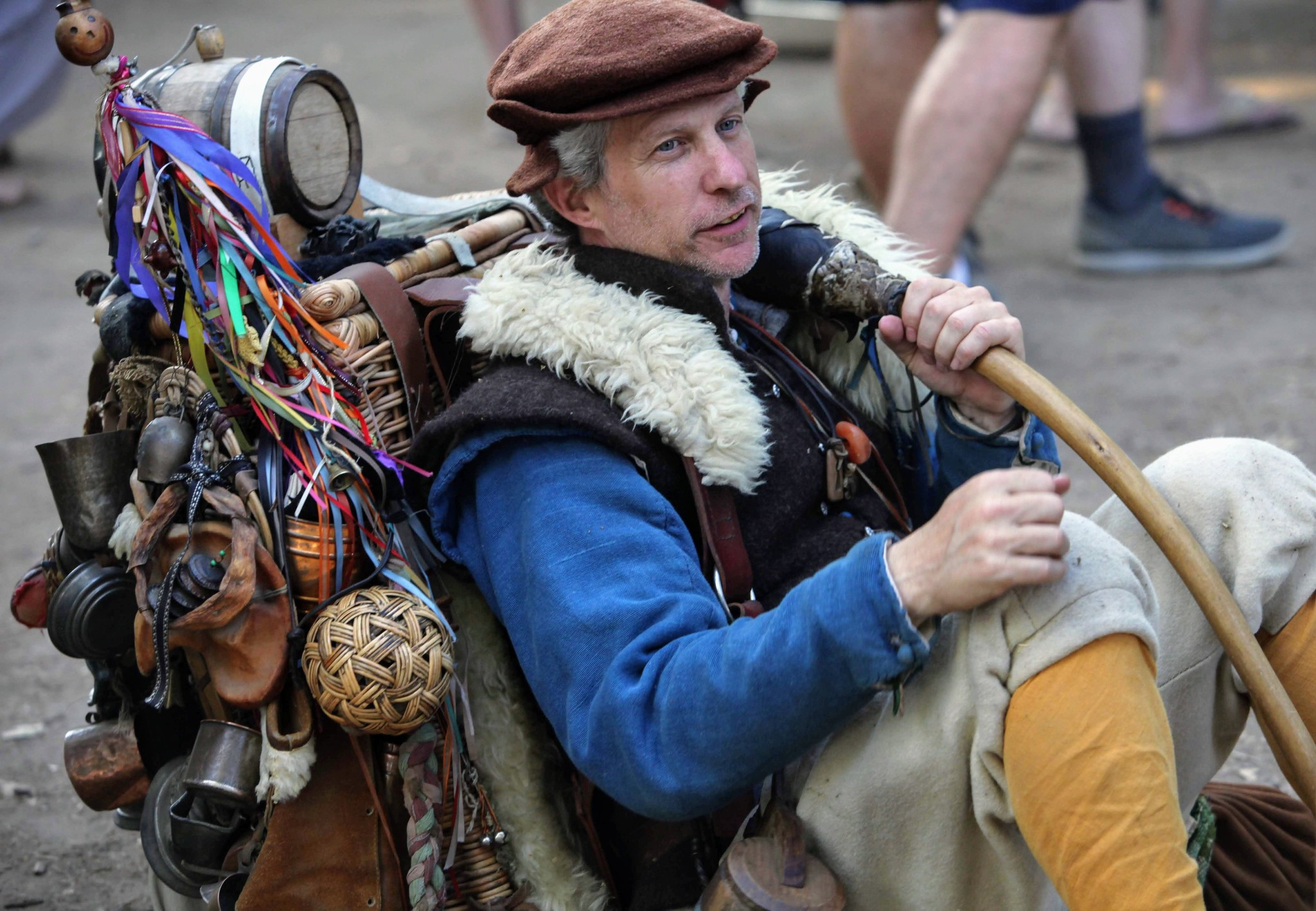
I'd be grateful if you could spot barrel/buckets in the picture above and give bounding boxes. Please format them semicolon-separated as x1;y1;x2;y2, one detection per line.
91;55;363;231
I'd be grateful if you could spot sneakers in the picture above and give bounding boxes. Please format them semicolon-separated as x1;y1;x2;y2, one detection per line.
1076;181;1293;270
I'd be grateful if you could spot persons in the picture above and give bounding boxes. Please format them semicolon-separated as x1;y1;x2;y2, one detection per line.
833;0;1301;303
401;0;1315;911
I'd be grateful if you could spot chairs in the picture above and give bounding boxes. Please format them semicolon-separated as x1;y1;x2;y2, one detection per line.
295;205;639;910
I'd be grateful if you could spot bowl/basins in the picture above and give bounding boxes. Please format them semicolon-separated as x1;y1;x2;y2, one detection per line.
47;554;137;658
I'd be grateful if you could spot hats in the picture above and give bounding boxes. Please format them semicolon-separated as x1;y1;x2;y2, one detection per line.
486;0;777;196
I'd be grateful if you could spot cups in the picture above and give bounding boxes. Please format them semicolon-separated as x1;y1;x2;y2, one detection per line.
61;715;158;812
288;515;359;606
181;718;270;803
32;423;135;553
